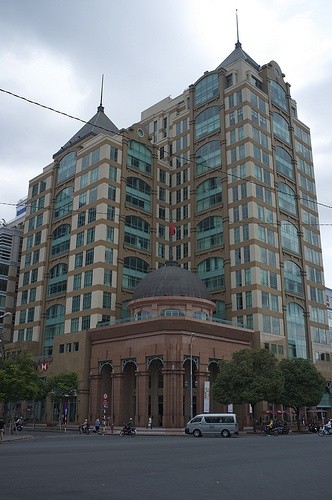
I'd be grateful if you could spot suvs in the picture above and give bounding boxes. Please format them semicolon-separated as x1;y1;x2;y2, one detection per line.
305;409;332;432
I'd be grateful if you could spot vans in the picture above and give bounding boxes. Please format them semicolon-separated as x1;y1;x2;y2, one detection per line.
185;413;239;439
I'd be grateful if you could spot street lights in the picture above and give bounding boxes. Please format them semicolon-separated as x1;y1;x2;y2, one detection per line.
189;333;197;419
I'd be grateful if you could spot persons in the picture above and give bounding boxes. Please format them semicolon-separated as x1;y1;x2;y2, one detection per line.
145;415;152;429
123;418;133;433
94;419;100;433
324;419;331;434
16;416;24;429
266;419;273;436
81;419;88;431
307;418;329;431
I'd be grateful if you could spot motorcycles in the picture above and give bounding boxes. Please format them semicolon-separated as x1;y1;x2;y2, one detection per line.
317;424;332;437
120;423;137;438
78;424;91;435
13;423;23;432
260;410;290;437
